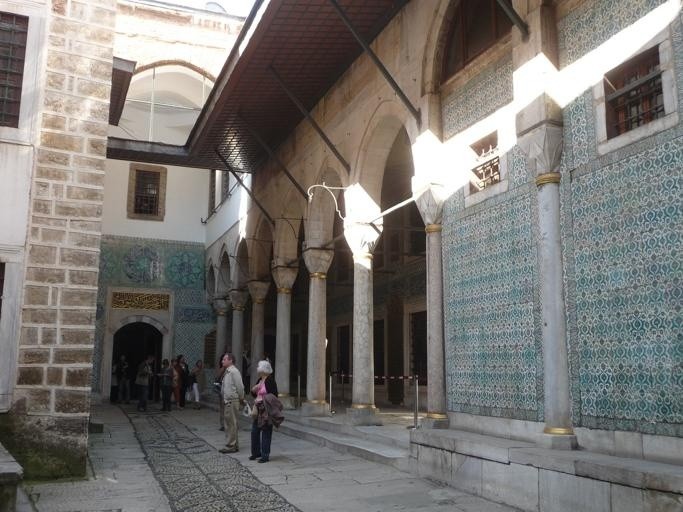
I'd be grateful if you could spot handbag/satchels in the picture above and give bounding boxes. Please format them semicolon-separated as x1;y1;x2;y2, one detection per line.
247;402;258;420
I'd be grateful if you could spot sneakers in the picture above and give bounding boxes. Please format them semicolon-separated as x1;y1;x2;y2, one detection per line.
117;399;201;412
219;427;224;431
218;447;269;463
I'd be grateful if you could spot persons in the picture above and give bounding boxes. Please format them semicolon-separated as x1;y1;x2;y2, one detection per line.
112;353;204;412
249;359;278;463
218;343;274;431
218;352;245;454
